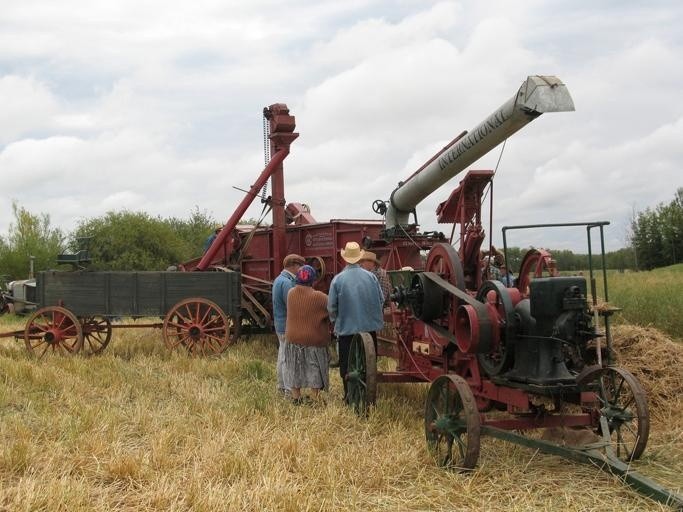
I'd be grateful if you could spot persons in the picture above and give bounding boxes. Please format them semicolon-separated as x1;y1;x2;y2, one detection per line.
205;225;223;252
500;263;516;288
480;255;500;281
327;241;384;418
357;251;392;314
280;265;330;406
271;253;305;401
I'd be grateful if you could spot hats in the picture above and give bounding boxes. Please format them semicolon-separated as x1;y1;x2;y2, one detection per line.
283;254;315;283
340;241;365;265
361;251;383;265
